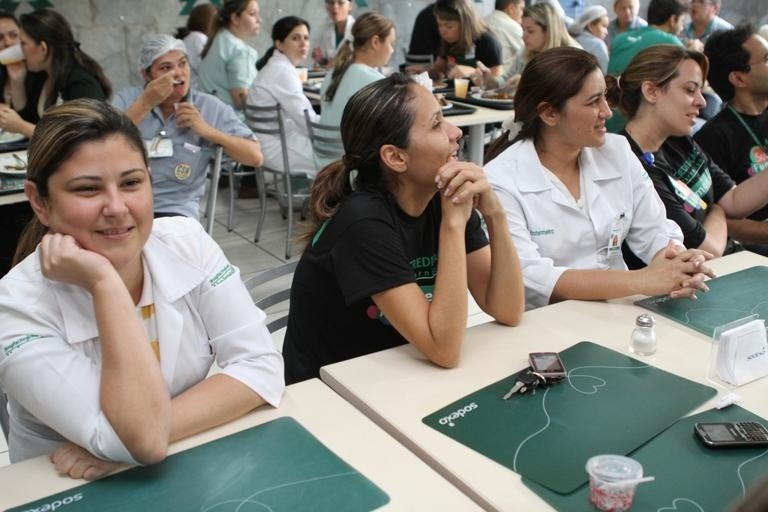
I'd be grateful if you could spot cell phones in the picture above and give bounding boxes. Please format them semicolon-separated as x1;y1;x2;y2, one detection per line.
694;422;768;449
529;352;566;380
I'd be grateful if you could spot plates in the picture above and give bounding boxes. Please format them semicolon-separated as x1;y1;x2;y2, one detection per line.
437;93;517;110
1;131;29;178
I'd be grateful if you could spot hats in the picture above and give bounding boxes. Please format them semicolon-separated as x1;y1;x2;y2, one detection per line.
137;32;189;73
578;5;608;30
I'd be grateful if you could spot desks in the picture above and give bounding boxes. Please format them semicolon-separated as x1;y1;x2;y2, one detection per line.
319;250;768;512
437;89;519;166
0;374;490;512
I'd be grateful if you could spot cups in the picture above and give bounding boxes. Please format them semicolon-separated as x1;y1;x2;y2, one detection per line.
173;102;192;128
585;454;644;512
453;78;469;99
298;67;308;82
0;43;27;66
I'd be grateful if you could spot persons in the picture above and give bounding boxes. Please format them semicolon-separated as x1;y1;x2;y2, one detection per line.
246;1;768;312
283;73;524;386
1;1;285;481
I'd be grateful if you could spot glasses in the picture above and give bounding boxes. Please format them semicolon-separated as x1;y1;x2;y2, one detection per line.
748;55;768;68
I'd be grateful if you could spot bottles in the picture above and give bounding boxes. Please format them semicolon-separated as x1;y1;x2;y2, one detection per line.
629;313;657;357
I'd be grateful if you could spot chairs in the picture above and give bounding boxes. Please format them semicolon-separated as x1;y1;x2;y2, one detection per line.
213;88;287;234
199;142;225;238
239;91;313;260
302;108;345;164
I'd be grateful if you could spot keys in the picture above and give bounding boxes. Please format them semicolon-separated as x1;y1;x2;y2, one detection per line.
503;367;538;400
